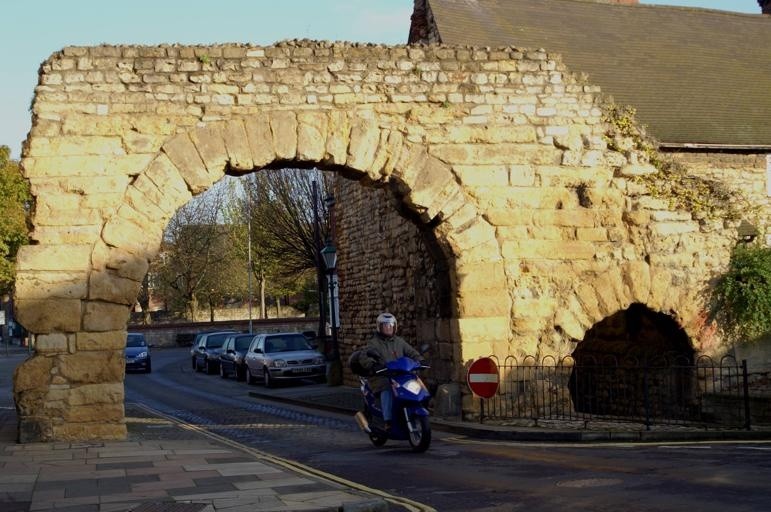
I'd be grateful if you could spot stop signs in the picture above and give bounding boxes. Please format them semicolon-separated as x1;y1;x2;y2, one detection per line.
466;358;502;397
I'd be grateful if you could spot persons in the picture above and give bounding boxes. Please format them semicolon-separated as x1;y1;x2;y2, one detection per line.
356;309;431;434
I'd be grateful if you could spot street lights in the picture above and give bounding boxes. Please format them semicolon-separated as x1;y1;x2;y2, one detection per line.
318;239;343;385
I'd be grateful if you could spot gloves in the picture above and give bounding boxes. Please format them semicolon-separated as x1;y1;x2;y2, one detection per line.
421;360;428;371
373;362;385;372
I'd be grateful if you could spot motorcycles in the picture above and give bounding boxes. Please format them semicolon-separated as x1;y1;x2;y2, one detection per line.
351;341;437;453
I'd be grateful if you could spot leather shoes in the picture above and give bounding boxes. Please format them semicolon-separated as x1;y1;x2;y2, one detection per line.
384;422;393;430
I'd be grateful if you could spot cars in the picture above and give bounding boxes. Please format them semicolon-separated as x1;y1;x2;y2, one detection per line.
124;331;155;376
191;326;329;385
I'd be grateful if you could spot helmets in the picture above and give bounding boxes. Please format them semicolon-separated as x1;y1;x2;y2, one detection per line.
376;312;399;334
